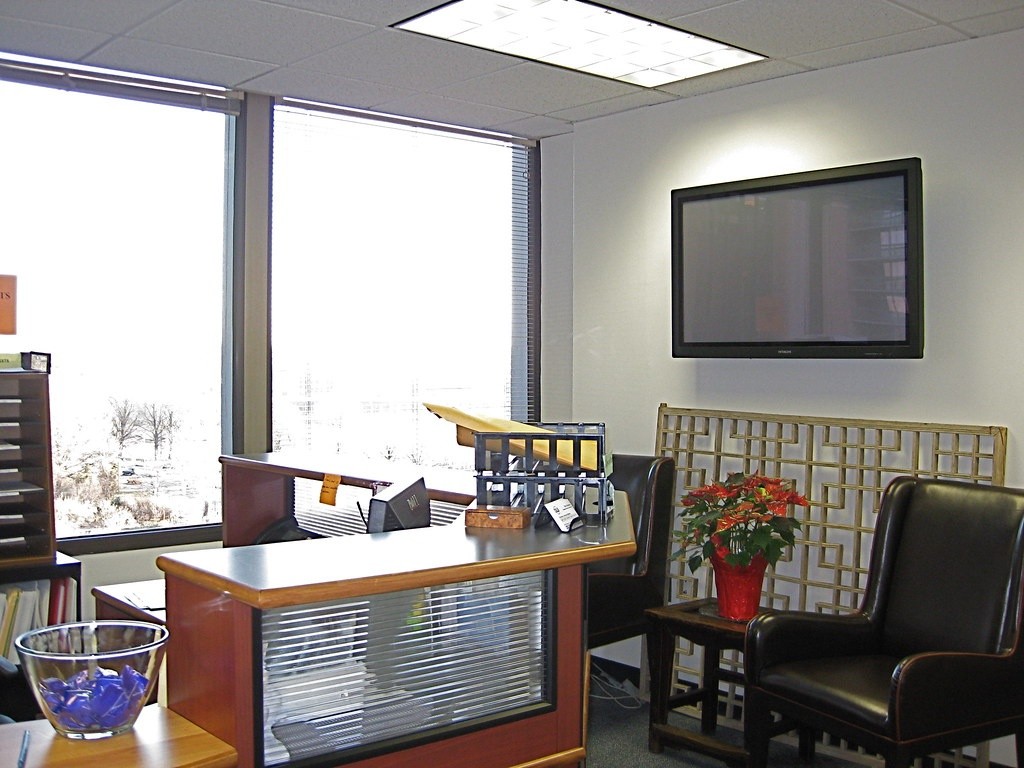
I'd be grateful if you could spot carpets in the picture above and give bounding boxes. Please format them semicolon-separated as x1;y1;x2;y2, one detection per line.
587;669;869;768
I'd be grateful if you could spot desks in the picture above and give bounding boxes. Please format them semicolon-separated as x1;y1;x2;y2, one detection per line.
639;596;787;768
0;450;647;768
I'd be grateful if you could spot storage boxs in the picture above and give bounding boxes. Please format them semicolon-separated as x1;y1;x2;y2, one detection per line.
463;505;533;530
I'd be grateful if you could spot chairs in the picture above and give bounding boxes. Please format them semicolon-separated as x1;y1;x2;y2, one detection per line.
742;471;1024;768
514;455;678;651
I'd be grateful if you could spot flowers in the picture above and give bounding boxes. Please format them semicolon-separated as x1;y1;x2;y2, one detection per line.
668;472;807;572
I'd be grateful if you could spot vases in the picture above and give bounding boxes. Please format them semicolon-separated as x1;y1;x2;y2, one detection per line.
706;551;770;622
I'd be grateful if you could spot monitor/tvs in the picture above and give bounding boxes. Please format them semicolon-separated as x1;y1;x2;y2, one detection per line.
671;158;925;360
366;476;430;533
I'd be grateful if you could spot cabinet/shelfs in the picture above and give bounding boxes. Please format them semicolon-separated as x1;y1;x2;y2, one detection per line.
0;372;57;570
0;550;82;670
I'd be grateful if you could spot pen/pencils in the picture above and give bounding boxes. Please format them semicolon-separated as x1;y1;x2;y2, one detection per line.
16;729;30;768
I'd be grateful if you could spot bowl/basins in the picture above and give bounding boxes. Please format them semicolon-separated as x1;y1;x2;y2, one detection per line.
15;620;169;740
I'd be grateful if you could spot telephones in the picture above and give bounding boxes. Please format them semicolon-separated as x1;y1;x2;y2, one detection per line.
248;514;328;546
